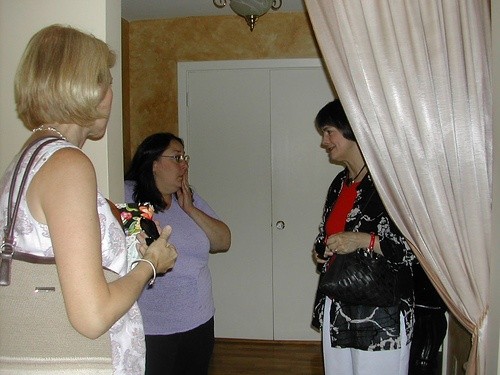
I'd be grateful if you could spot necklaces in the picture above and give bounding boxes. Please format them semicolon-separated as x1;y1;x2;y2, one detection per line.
346;164;366;186
32;126;66;142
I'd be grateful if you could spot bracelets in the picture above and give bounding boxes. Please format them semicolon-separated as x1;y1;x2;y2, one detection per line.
368;230;376;251
130;259;157;287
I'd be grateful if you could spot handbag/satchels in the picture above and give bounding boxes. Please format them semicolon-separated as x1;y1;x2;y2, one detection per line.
321;252;400;309
0;135;121;375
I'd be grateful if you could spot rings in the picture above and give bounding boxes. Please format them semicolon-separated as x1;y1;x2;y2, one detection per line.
334;249;337;254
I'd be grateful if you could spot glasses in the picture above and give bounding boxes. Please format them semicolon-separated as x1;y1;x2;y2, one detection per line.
159;154;190;163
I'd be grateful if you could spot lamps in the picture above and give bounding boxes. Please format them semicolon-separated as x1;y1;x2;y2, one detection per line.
212;0;282;31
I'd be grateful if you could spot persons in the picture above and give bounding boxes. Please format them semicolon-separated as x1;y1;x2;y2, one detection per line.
0;25;179;375
313;98;448;375
124;132;231;375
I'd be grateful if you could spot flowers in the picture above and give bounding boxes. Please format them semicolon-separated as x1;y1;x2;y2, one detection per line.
116;202;154;240
